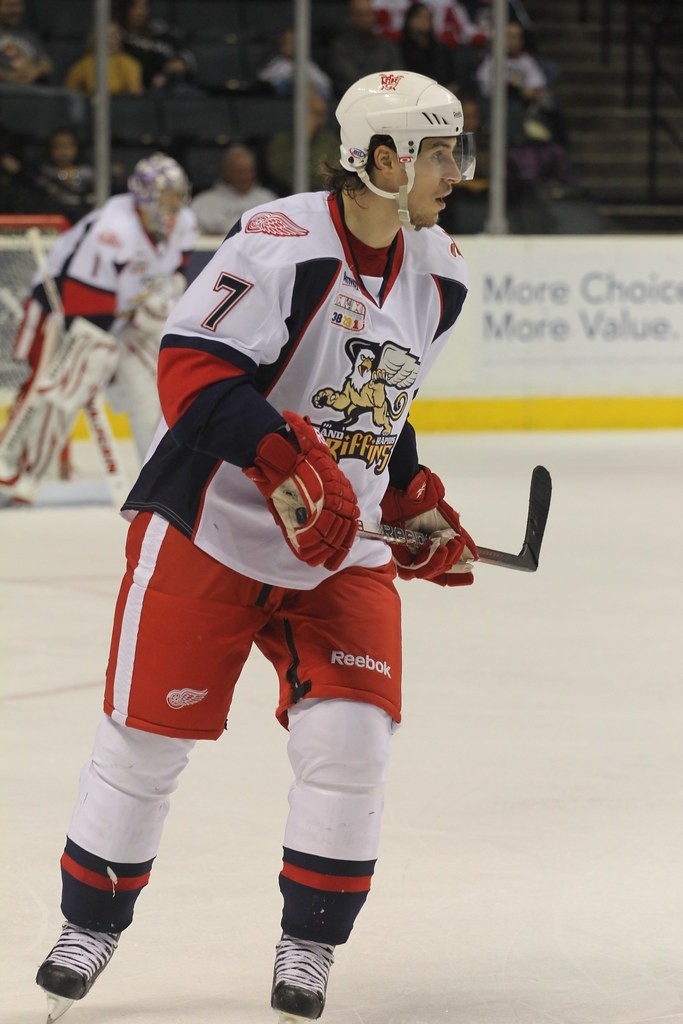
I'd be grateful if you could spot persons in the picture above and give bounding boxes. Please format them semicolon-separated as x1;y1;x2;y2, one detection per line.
0;156;200;508
0;0;594;237
188;143;281;235
37;71;481;1024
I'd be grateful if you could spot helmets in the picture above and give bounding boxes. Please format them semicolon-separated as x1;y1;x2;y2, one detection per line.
335;70;476;173
127;152;191;230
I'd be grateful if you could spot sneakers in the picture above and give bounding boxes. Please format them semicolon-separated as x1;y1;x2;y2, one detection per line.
270;931;336;1024
36;919;121;1024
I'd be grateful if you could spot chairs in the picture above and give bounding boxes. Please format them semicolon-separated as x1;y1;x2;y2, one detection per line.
0;0;515;237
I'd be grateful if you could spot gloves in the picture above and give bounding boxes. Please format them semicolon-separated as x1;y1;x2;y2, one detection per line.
241;410;360;571
380;464;479;587
131;272;186;334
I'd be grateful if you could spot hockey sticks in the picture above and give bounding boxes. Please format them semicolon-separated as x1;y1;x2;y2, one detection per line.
294;464;552;573
25;227;131;506
